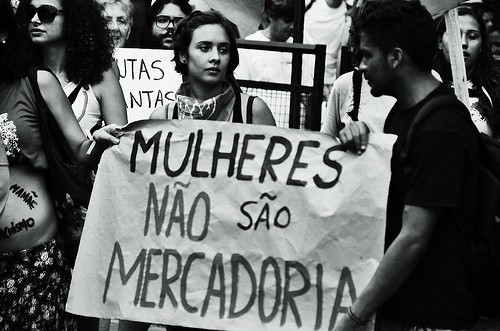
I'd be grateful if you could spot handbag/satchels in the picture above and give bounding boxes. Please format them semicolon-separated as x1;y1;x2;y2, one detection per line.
27;66;94;260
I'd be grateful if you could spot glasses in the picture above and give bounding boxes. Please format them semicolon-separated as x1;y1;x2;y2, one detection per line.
153;16;183;29
21;4;65;24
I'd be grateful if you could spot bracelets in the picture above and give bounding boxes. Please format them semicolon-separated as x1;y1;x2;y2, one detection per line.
348;306;369;325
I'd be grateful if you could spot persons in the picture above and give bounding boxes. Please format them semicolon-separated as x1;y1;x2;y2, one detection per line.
16;0;127;269
429;6;500;140
304;0;351;126
0;0;132;331
148;0;195;51
244;1;295;43
149;9;277;126
333;0;500;331
320;69;397;155
95;0;134;49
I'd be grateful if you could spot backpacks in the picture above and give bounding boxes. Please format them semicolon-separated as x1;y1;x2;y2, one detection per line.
399;97;500;322
